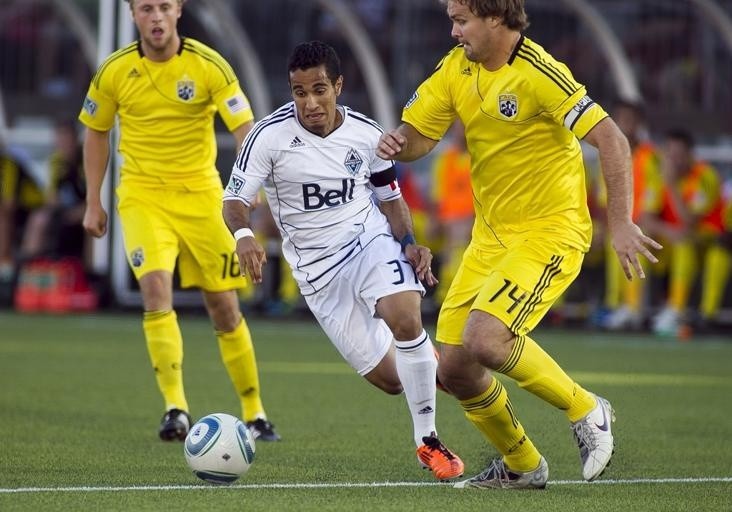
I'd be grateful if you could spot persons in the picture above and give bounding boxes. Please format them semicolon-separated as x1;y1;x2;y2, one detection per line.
22;116;91;256
372;1;668;492
399;103;728;334
0;146;48;310
74;2;280;443
221;41;465;480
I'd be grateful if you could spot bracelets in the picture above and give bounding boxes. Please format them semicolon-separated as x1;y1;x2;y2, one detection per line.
232;225;260;246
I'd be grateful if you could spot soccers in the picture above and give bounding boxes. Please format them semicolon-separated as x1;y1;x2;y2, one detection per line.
184;413;256;484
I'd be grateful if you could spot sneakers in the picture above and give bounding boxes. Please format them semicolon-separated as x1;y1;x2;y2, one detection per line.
415;431;466;482
453;454;549;492
158;408;194;442
245;416;282;443
570;395;618;483
